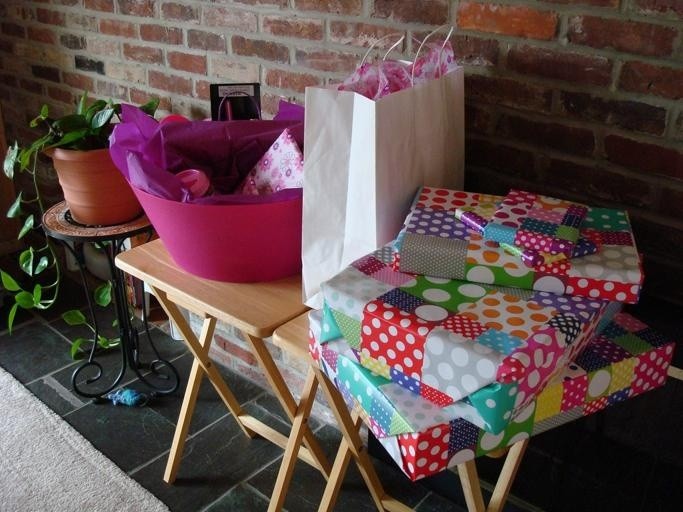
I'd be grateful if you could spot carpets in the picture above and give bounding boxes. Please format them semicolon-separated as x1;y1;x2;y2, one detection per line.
0;367;170;512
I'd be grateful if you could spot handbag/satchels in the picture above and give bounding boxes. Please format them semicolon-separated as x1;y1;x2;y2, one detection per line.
300;24;467;311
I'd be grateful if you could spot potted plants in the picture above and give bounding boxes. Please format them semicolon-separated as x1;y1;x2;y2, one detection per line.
0;85;159;353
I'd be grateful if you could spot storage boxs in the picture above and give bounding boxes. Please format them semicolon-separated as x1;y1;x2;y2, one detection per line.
396;185;647;303
297;306;675;480
323;246;628;430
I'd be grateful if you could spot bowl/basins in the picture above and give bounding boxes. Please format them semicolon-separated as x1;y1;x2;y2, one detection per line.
125;113;302;283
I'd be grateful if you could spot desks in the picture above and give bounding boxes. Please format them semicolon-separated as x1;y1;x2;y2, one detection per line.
267;310;536;512
43;199;182;398
112;234;328;512
